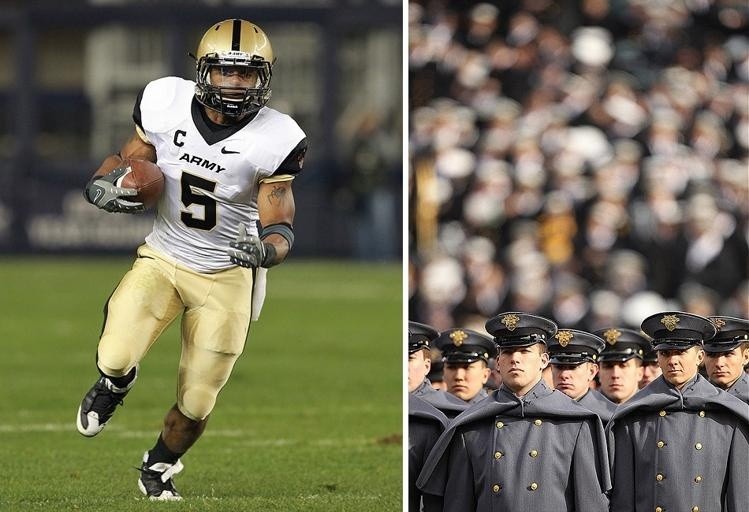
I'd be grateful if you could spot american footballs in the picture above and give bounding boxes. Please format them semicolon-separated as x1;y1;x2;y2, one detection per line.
115;159;165;209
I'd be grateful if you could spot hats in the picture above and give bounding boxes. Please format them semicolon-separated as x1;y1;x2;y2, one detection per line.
408;311;749;366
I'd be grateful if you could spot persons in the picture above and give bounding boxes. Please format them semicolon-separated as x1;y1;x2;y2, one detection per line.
408;312;748;512
75;16;309;501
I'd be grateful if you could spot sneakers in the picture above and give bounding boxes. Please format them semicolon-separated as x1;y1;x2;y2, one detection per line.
77;375;137;437
136;450;183;501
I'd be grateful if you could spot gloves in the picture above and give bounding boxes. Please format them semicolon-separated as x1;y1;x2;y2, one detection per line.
83;166;145;214
227;222;268;268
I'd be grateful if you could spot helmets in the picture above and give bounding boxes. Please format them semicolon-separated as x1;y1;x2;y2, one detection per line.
189;19;277;117
